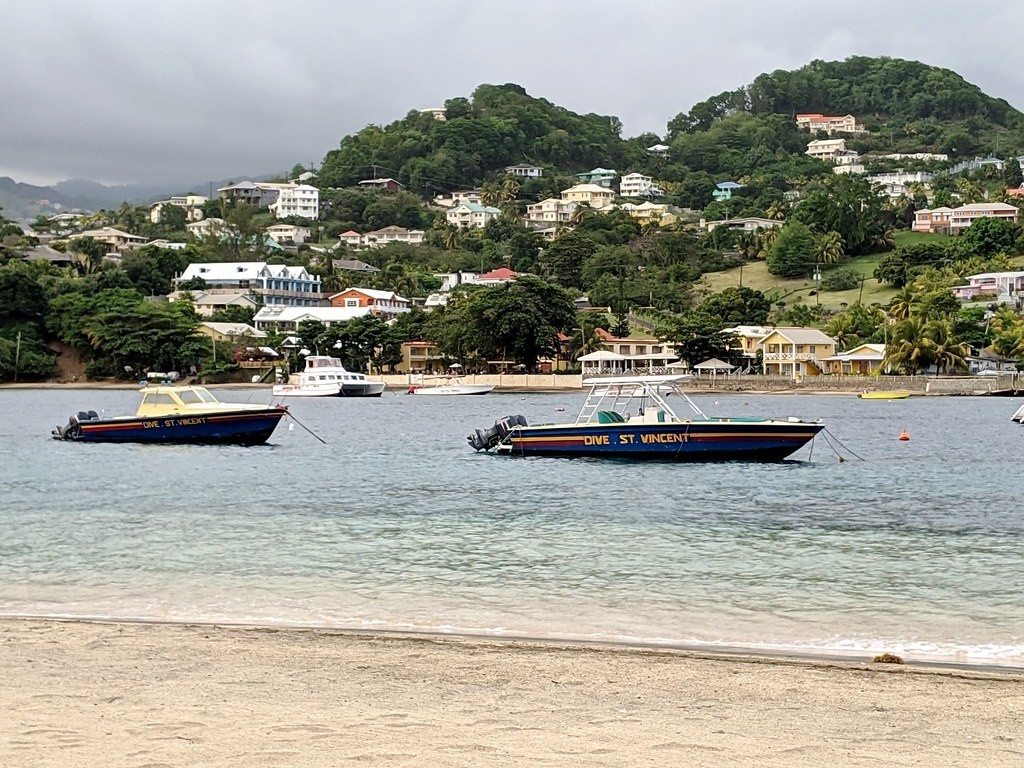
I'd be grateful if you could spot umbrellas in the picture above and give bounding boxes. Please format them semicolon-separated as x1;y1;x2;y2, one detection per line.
449;363;462;375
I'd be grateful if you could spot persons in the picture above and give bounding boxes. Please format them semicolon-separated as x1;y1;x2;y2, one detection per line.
408;386;416;395
282;376;286;384
382;367;484;375
624;407;644;423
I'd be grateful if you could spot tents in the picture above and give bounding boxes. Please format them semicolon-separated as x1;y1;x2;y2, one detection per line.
626;352;680;374
694;358;735;380
578;351;625;377
667;360;691;374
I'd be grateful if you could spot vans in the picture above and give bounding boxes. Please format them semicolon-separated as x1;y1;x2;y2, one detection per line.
246;346;280;362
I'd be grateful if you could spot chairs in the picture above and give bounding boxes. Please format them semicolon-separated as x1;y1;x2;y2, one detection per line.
598;411;624;423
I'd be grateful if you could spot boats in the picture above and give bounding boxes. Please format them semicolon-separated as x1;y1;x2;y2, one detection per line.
272;350;385;397
414;373;496;395
465;374;827;462
48;371;291;446
857;388;911;398
